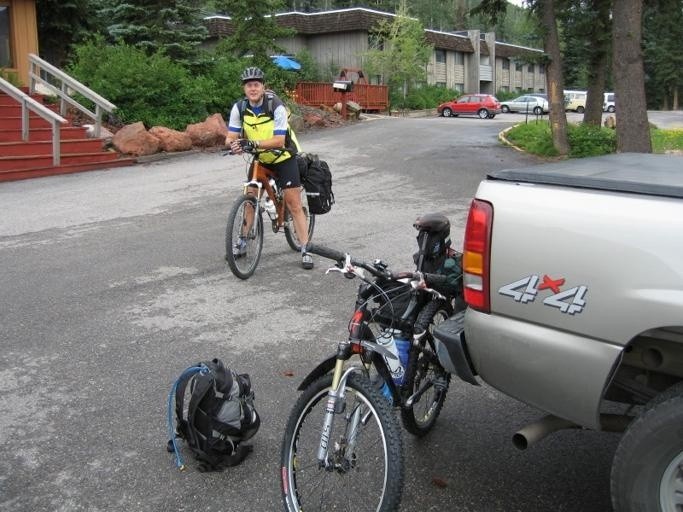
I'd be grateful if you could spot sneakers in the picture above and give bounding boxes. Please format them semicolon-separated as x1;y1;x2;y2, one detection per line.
225;242;247;260
302;251;314;270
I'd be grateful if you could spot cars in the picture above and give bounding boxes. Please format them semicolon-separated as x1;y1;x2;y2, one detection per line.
432;154;683;511
437;90;615;119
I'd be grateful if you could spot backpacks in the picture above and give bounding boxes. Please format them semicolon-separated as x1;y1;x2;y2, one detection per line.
296;152;335;215
166;358;261;473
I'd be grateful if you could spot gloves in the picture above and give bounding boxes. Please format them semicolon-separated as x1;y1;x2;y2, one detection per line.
238;138;258;151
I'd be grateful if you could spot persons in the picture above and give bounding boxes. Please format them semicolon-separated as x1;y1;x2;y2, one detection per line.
410;211;462;302
223;67;314;271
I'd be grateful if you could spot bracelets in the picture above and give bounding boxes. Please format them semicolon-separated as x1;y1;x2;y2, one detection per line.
254;140;259;148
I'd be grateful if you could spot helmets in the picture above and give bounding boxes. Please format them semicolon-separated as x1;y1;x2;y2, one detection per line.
240;66;265;83
